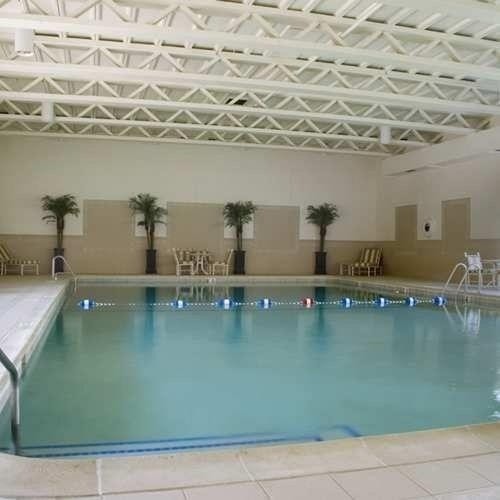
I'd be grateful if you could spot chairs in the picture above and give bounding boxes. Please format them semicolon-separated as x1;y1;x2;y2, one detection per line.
173;245;235;275
464;250;496;288
338;246;385;279
0;240;40;277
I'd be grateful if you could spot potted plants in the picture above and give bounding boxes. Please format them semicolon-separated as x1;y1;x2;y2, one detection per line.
40;192;80;273
220;200;259;276
303;202;339;276
127;192;171;274
